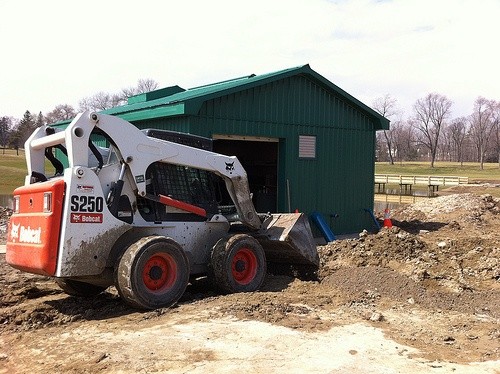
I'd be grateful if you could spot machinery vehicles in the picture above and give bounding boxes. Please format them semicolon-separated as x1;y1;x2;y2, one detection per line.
5;110;321;312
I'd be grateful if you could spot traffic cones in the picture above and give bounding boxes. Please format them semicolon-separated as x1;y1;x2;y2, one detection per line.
383;208;392;227
